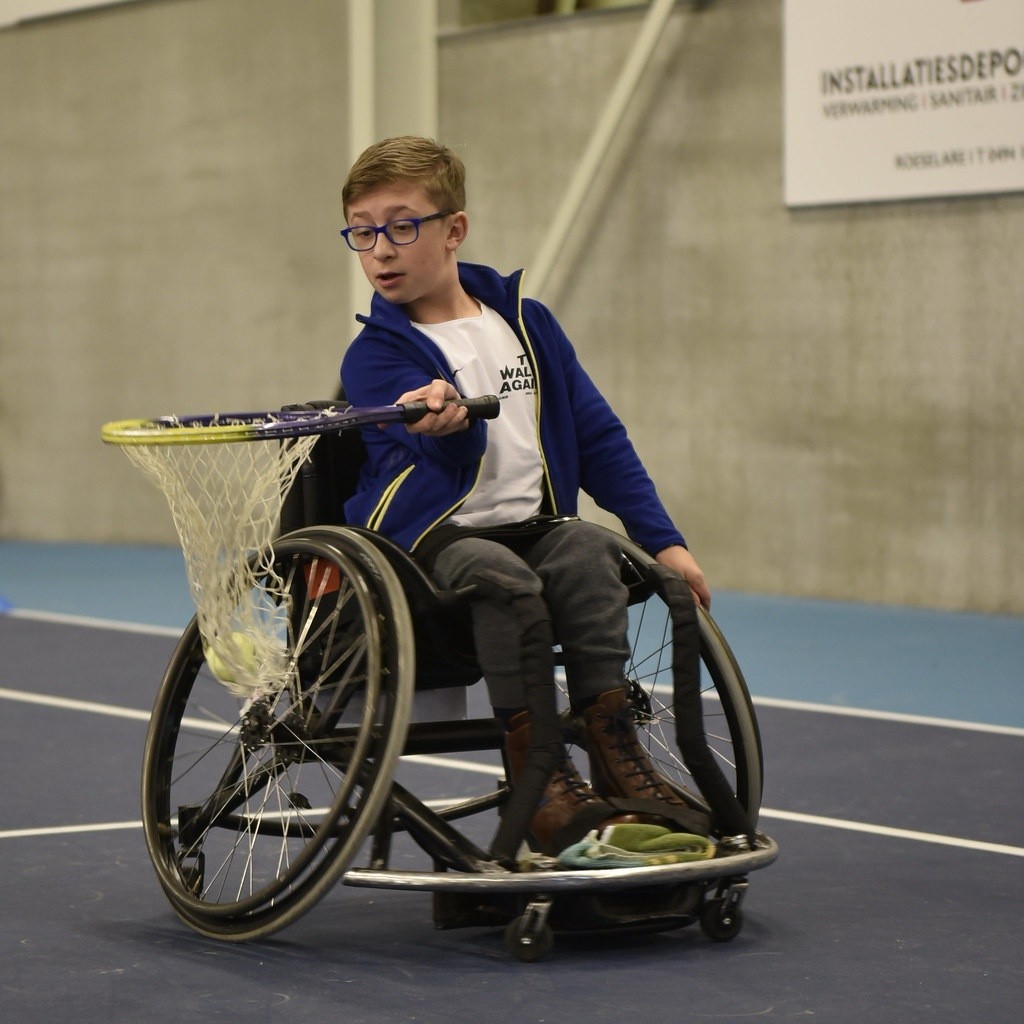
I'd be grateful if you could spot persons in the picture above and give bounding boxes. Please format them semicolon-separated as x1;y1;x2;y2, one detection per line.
340;134;712;855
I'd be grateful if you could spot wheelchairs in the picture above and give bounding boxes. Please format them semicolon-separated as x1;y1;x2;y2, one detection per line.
140;433;780;962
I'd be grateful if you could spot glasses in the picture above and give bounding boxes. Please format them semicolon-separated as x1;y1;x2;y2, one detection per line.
340;208;454;251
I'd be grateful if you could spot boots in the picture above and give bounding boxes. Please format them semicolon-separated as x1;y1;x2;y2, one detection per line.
572;687;690;827
494;707;640;857
